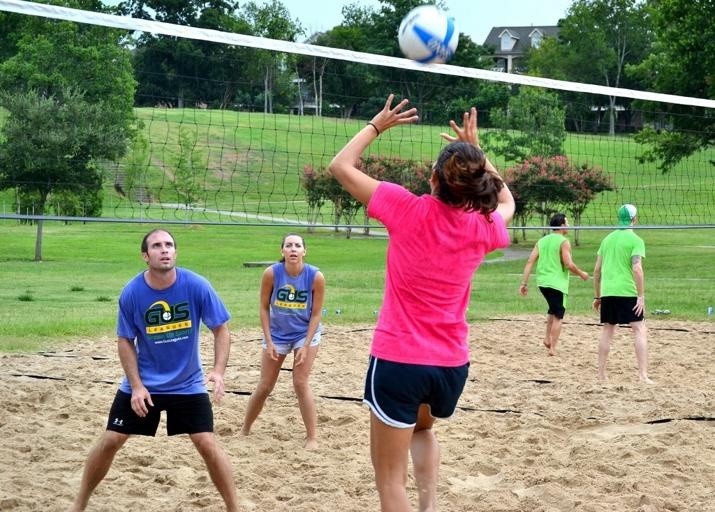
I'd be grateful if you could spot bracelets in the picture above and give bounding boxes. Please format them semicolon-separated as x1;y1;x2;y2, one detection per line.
367;123;379;136
521;284;527;286
594;298;601;299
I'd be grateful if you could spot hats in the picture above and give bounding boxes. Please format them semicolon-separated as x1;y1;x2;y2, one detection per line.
618;204;637;229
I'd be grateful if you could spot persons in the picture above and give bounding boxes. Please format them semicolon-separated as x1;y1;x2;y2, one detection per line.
66;228;243;512
591;204;655;386
234;232;327;454
518;213;589;357
328;92;518;512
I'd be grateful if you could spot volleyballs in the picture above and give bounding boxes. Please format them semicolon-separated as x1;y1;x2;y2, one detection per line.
398;3;459;65
